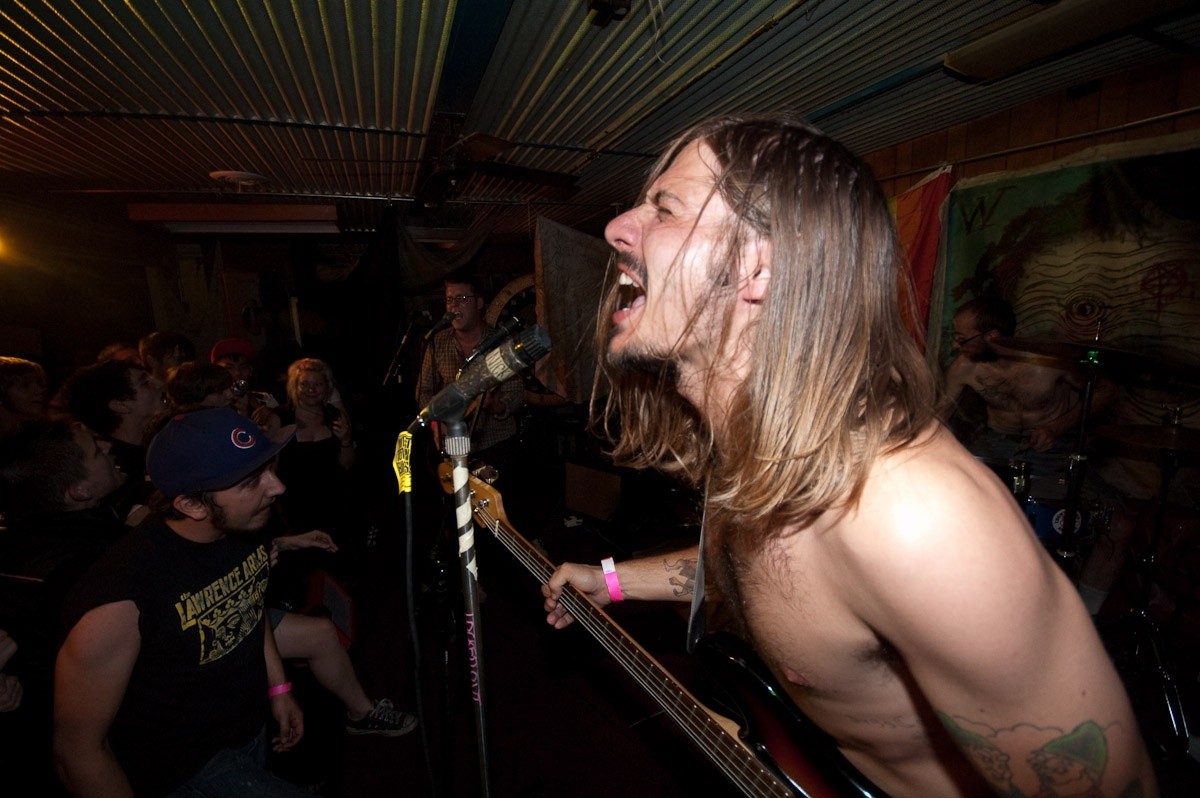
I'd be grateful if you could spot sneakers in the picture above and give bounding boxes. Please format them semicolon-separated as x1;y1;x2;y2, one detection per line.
344;698;418;737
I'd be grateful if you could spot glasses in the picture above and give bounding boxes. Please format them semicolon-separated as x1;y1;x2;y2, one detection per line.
443;294;484;304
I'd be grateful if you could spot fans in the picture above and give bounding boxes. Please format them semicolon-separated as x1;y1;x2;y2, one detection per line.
299;131;585;220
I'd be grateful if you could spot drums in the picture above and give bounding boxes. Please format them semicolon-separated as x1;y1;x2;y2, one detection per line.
1010;464;1115;584
941;430;1029;484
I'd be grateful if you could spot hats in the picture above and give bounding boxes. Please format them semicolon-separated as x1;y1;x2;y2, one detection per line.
144;408;297;500
210;337;255;365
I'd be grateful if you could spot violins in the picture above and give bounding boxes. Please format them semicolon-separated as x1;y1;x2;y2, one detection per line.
1136;544;1183;761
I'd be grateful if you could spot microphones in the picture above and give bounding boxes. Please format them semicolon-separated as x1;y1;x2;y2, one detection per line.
460;316;522;369
423;311;456;341
408;324;551;433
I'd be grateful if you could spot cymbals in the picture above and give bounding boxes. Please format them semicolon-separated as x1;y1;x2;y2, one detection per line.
1093;424;1200;455
988;332;1200;385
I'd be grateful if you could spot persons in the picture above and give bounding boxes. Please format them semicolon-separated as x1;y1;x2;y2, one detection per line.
414;270;526;576
542;108;1160;798
0;327;420;798
52;407;307;798
933;294;1136;620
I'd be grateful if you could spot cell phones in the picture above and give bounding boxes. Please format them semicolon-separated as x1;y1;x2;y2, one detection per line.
322;408;343;433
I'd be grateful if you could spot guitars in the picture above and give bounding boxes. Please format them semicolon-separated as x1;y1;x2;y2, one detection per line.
431;388;491;453
439;461;891;798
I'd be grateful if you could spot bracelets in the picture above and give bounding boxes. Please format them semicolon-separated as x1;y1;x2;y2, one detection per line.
268;682;292;699
601;557;624;603
